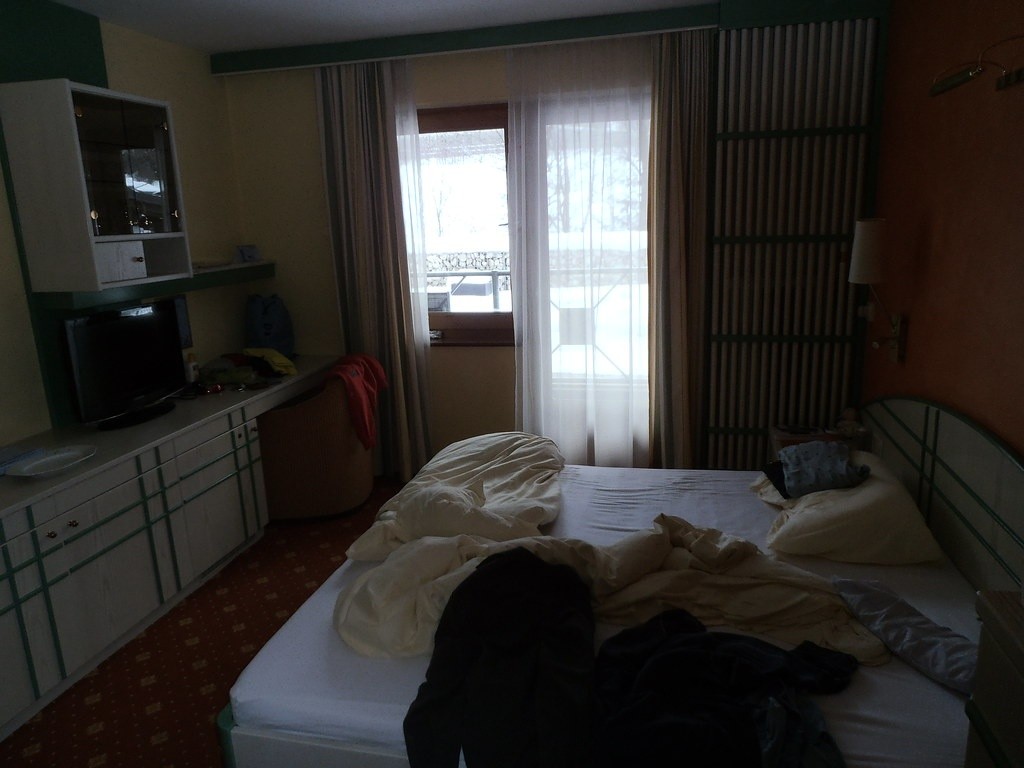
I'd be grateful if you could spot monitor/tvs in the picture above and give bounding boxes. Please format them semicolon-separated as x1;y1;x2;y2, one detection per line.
65;297;188;432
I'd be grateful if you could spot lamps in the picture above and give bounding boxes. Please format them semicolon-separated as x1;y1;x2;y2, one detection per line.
849;218;909;364
928;33;1024;98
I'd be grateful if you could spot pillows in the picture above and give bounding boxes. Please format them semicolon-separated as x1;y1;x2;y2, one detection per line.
749;451;943;565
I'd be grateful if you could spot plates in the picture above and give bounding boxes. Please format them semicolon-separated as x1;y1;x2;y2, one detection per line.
5;445;97;478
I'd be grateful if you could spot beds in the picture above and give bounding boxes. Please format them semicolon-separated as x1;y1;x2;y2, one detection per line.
218;394;1023;768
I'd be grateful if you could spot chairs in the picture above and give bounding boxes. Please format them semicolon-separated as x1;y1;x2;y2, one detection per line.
257;352;390;519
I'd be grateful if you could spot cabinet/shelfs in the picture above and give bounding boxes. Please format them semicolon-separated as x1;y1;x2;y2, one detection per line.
0;406;269;743
965;591;1024;768
0;78;193;292
